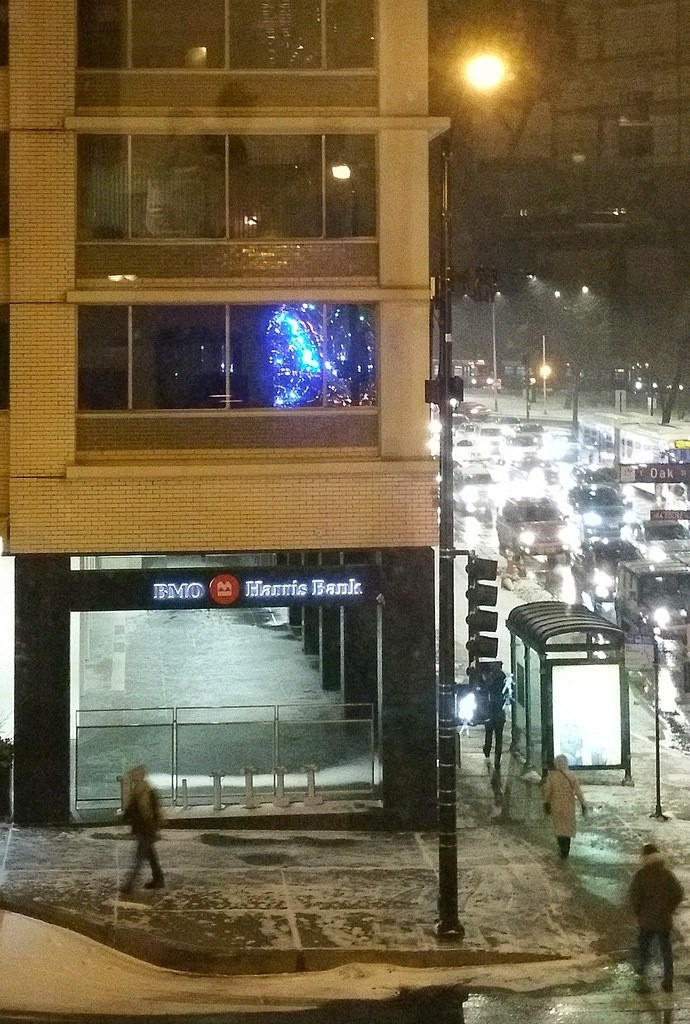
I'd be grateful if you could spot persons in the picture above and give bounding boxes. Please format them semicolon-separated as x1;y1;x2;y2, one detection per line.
481;666;509;757
119;765;165;892
629;843;683;995
544;756;587;860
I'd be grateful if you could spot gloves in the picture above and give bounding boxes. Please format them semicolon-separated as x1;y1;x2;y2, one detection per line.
581;804;587;817
543;803;550;815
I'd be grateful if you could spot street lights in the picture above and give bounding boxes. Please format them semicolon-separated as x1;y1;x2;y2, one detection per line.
434;51;510;943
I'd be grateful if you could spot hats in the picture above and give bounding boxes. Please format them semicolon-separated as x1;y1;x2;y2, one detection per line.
131;766;144;781
555;754;568;770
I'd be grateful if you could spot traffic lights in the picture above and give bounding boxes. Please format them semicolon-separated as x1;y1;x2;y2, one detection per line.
465;552;501;659
451;685;484;724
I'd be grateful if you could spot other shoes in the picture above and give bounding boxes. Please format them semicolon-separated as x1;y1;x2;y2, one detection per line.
634;979;648;993
118;884;132;893
495;764;500;770
145;879;165;889
483;748;490;757
661;978;673;993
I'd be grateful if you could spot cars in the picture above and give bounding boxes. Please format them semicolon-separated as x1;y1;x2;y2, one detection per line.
454;404;690;639
